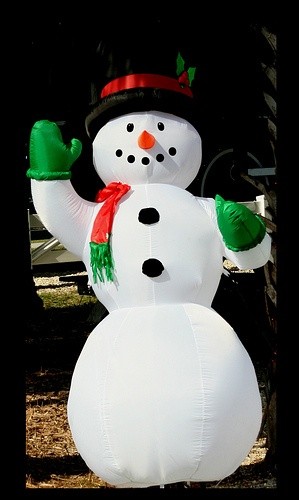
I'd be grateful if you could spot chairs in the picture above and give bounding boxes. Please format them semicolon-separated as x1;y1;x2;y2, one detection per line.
27;209;91;294
